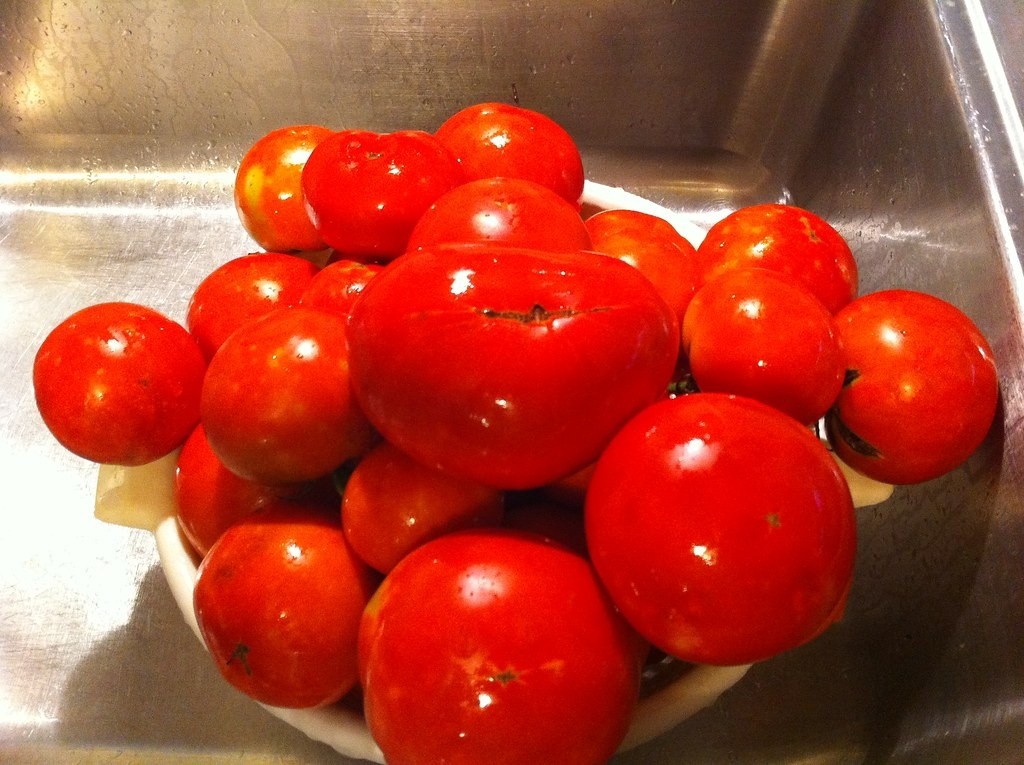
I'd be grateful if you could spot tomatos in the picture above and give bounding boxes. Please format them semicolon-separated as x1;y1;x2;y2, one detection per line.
33;103;1000;765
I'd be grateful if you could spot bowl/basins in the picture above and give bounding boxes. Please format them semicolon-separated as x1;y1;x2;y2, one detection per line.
146;173;816;765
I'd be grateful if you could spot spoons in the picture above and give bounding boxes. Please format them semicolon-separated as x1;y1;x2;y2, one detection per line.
814;436;904;517
